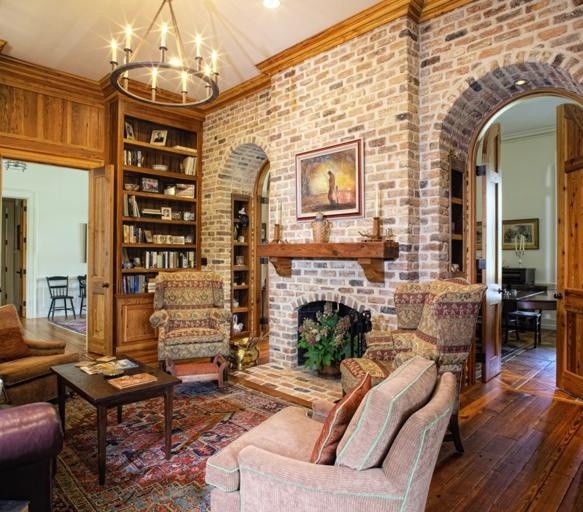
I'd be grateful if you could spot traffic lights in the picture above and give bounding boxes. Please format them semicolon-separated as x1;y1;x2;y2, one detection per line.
310;372;371;465
0;324;30;361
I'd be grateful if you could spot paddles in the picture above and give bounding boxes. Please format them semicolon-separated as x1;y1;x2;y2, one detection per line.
48;318;86;336
41;373;312;512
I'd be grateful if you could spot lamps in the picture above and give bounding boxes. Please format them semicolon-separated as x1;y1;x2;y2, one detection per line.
46;276;76;321
503;298;521;343
340;278;488;460
78;274;87;316
150;270;232;388
508;286;546;347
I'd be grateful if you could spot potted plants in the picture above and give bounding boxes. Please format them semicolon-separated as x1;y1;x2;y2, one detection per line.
125;120;135;139
175;183;195;198
500;218;539;249
144;229;153;243
160;207;171;220
296;139;363;219
150;127;168;146
140;176;160;193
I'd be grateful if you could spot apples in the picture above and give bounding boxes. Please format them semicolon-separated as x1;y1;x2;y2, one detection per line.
274;199;279;224
374;186;380;217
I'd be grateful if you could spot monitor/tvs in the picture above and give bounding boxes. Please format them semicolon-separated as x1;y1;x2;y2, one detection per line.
108;373;158;389
141;251;196;268
124;149;143;167
183;157;197;175
123;225;194;245
122;273;156;293
123;193;162;218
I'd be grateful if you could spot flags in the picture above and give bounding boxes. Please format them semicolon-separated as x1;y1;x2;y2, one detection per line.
321;364;340;377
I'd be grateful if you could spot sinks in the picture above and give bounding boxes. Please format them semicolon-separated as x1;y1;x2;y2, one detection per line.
205;355;457;512
0;402;64;512
0;304;79;406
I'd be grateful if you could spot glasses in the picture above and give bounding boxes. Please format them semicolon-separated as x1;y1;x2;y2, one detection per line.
110;0;219;107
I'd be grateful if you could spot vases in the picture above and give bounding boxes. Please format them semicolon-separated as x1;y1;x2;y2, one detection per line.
298;300;351;366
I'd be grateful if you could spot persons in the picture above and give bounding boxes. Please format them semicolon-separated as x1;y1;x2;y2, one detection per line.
153;132;163;143
327;170;336;203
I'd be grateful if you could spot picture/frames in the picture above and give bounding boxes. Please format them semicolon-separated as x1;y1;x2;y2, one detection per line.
506;289;558;343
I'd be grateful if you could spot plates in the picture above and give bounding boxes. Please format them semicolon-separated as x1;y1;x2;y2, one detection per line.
110;114;206;363
499;269;534;332
231;193;252;340
